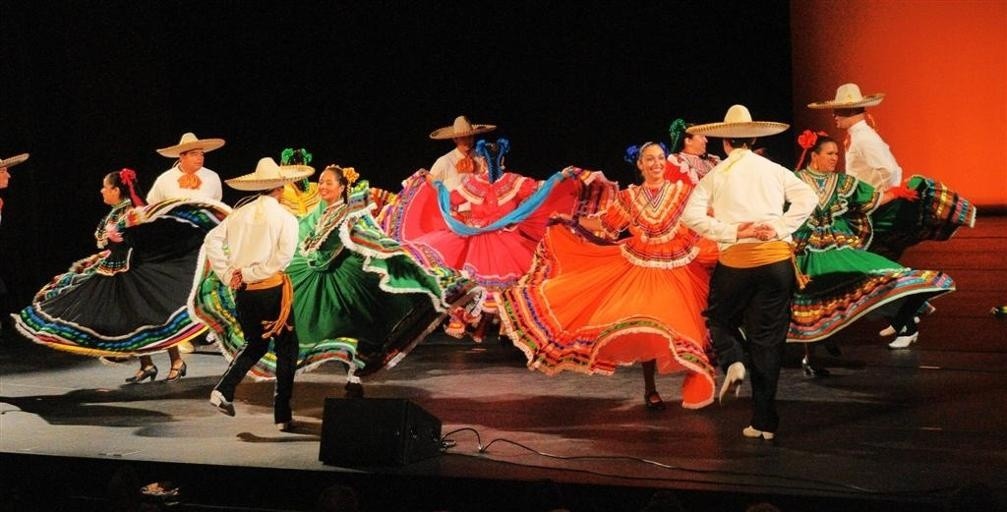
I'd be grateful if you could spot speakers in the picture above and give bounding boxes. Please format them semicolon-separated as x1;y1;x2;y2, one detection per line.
319;398;441;468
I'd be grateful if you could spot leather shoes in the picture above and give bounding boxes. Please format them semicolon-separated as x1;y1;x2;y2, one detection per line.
644;317;920;440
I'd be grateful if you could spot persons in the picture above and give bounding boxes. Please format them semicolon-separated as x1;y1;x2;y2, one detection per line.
492;141;720;411
145;133;226;353
666;118;722;186
427;115;497;179
204;157;316;430
677;103;817;442
375;138;620;345
276;147;323;218
9;168;234;385
805;82;920;349
787;128;978;382
186;164;488;401
0;152;30;210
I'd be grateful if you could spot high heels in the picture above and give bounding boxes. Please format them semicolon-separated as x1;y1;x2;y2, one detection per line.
127;341;297;431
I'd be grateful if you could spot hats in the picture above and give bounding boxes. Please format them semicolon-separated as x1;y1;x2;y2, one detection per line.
225;157;316;191
429;116;497;140
686;105;790;138
0;152;30;168
155;133;226;158
808;84;885;109
